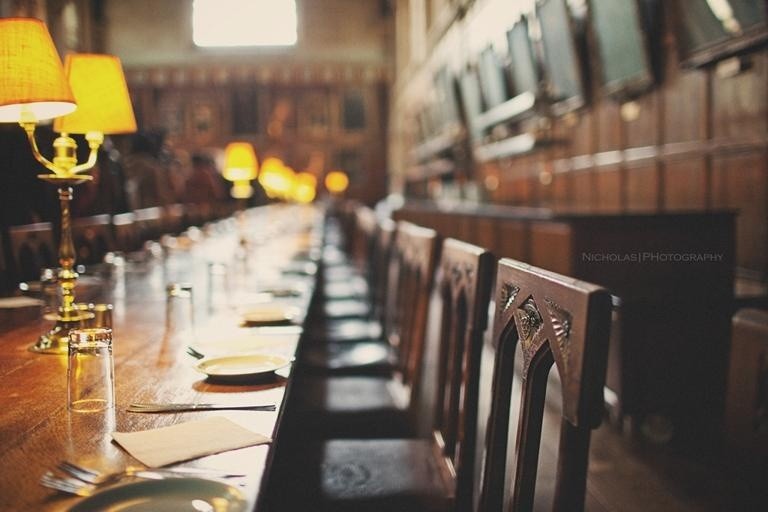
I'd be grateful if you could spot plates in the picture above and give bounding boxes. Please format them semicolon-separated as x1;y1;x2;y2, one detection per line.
64;475;253;512
193;349;292;384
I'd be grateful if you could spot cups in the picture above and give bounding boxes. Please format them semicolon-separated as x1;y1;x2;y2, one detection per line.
63;321;117;414
36;203;310;335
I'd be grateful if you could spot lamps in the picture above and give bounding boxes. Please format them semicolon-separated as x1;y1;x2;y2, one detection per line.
1;16;138;356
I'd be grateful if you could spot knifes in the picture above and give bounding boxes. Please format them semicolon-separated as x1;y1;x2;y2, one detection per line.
123;399;278;418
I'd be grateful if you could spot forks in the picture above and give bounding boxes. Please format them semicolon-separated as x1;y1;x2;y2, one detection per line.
34;454;254;497
185;343;207;361
